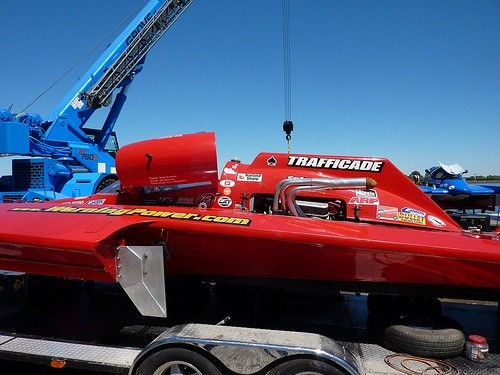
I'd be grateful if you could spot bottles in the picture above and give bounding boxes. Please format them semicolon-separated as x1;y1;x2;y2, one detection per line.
465;335;489;362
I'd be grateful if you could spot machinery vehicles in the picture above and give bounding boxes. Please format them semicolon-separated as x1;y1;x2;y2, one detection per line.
1;0;363;374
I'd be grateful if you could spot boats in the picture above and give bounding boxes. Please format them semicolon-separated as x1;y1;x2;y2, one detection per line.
411;162;495;199
0;130;500;320
